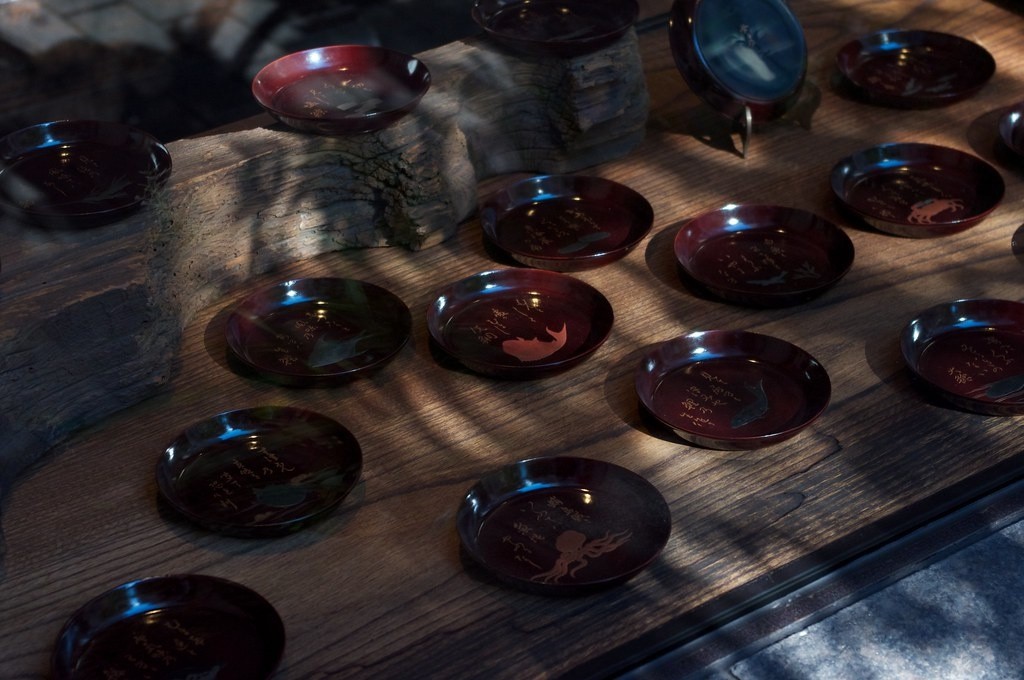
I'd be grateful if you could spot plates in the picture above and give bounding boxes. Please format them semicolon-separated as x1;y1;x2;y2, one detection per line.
830;142;1005;239
250;44;431;135
633;329;832;451
456;455;672;598
899;297;1024;416
223;277;413;389
154;406;364;540
50;573;286;680
835;29;996;111
999;102;1024;158
0;118;173;231
426;267;614;383
673;205;855;310
668;0;808;126
470;1;640;58
478;174;655;273
